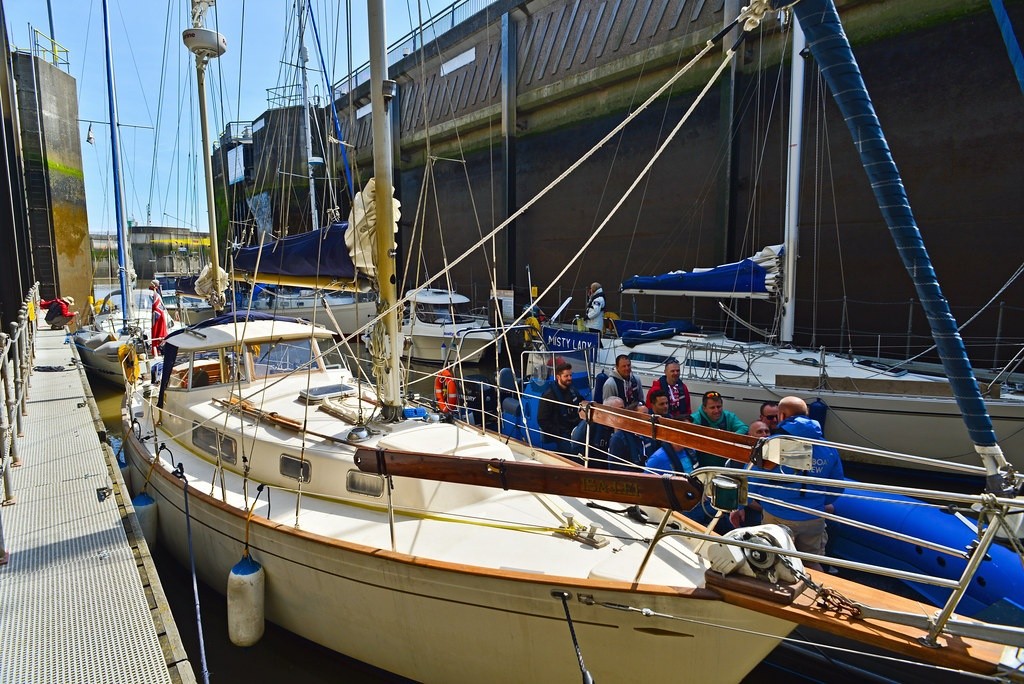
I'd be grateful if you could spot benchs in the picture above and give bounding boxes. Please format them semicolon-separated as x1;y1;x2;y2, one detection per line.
499;371;592;452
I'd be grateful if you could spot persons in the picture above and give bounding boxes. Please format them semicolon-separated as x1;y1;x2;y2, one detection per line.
606;401;646;473
572;396;624;470
40;296;80;330
586;281;605;349
691;391;748;436
647;360;691;419
537;363;586;441
642;415;699;477
602;355;644;410
744;395;845;574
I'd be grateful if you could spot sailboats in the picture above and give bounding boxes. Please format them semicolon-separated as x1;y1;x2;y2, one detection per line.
0;0;1024;684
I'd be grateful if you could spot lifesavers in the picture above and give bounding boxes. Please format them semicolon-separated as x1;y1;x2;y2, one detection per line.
434;369;458;412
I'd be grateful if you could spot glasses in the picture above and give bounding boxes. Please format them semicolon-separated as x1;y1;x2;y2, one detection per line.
665;358;680;366
704;393;722;403
761;413;778;420
636;401;643;407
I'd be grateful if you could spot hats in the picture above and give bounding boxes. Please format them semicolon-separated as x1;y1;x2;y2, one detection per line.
62;296;75;305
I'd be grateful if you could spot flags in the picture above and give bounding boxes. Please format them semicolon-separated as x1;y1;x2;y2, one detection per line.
87;124;95;146
151;285;168;356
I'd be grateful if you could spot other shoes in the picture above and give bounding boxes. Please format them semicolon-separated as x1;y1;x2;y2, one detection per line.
51;325;64;330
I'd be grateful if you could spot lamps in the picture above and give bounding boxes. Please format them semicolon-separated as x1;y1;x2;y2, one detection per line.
381;80;398;114
